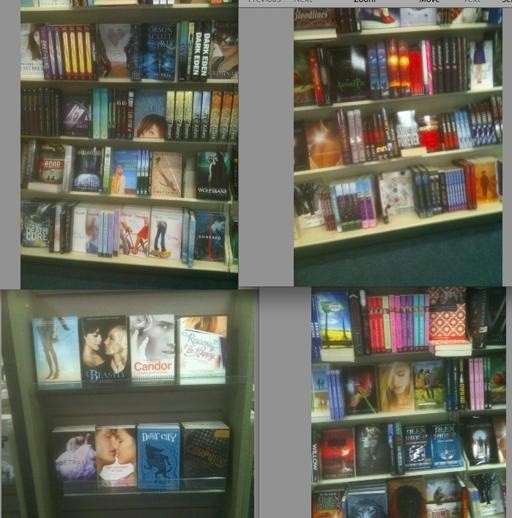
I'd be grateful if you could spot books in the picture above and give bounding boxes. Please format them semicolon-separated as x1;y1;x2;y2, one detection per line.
95;85;166;138
379;362;415;412
138;423;182;490
185;423;231;493
154;152;235;200
169;85;240;142
355;427;387;476
404;426;429;470
323;425;356;478
311;484;348;518
348;480;386;518
21;85;93;137
21;23;98;82
448;359;508;413
130;315;179;388
349;290;431;355
292;108;403;169
388;36;497;96
181;316;232;385
431;287;506;356
368;5;436;27
430;426;462;469
440;7;501;26
72;147;151;196
21;0;242;7
413;362;446;409
20;140;72;193
99;425;138;493
309;423;322;482
379;158;501;216
294;9;364;36
82;313;130;389
54;425;96;493
132;23;240;82
20;199;119;258
425;474;460;517
97;23;133;80
34;313;79;392
293;179;326;230
387;477;425;518
320;173;381;232
456;469;507;518
451;414;499;466
387;421;405;476
120;204;239;268
310;291;356;363
397;94;506;153
311;364;377;419
294;43;390;105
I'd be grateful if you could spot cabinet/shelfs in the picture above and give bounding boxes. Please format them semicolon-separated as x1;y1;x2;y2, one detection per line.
18;0;238;275
295;6;504;259
313;288;510;513
3;288;256;518
